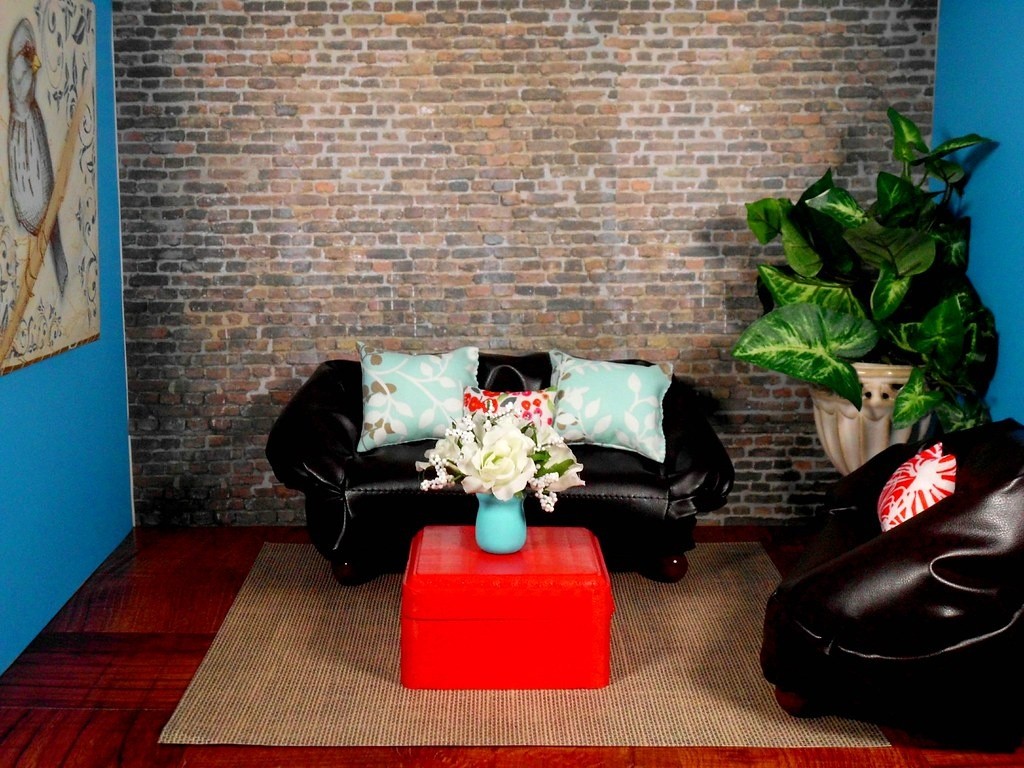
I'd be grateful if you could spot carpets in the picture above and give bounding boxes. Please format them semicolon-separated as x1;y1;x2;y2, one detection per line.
157;541;889;746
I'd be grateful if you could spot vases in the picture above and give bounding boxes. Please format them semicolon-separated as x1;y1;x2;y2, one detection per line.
474;489;521;554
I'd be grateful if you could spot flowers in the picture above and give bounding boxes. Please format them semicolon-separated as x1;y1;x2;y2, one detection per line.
416;413;572;512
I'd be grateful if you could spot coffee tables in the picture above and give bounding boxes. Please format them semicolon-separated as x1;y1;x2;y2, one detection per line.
400;527;614;689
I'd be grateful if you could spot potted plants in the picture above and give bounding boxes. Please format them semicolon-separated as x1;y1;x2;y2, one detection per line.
731;106;999;477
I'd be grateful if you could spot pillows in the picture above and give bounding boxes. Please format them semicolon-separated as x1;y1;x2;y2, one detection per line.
548;347;674;463
877;443;958;533
357;341;479;455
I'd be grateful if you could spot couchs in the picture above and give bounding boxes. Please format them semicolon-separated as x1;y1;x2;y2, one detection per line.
758;418;1024;754
266;351;735;583
